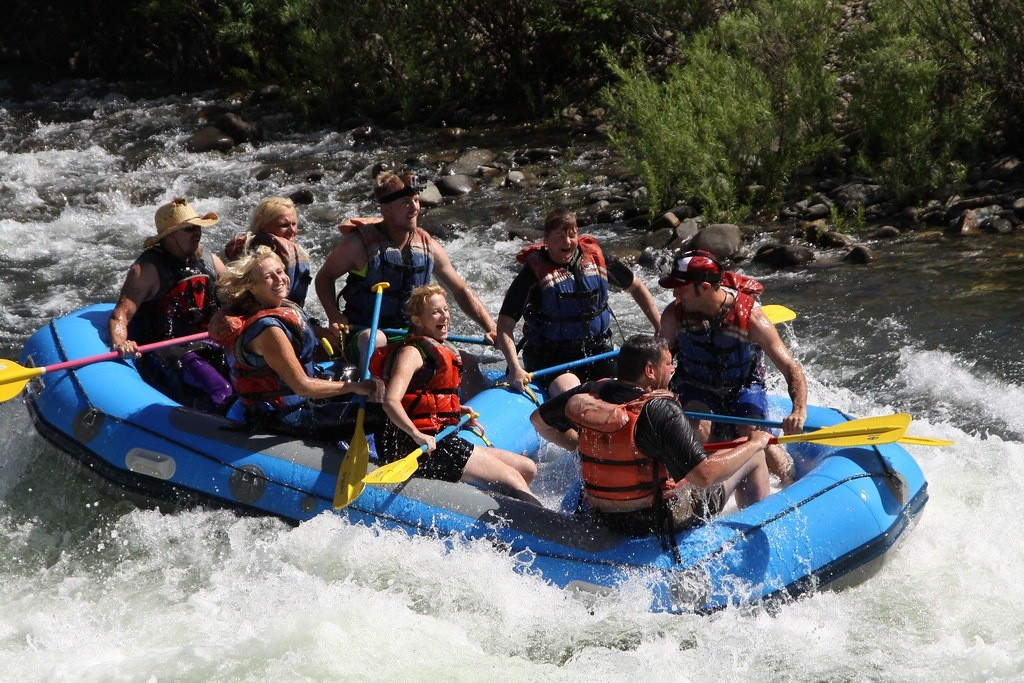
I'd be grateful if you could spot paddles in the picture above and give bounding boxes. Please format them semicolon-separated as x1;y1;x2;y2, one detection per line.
682;410;952;448
333;281;392;513
701;411;912;452
520;304;797;387
335;322;524;350
360;410;482;485
0;330;210;404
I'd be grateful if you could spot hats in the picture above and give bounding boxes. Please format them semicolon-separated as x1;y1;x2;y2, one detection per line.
658;249;725;288
142;198;219;249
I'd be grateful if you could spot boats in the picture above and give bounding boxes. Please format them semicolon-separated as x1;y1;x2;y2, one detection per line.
19;303;928;619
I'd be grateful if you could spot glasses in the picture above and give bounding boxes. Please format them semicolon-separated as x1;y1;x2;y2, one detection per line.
181;225;202;233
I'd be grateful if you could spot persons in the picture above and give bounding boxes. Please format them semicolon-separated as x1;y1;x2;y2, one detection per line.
373;285;543;508
108;200;238;417
658;248;807;484
216;245;373;443
315;171;496;380
530;334;776;537
497;208;662;399
231;197;309;309
